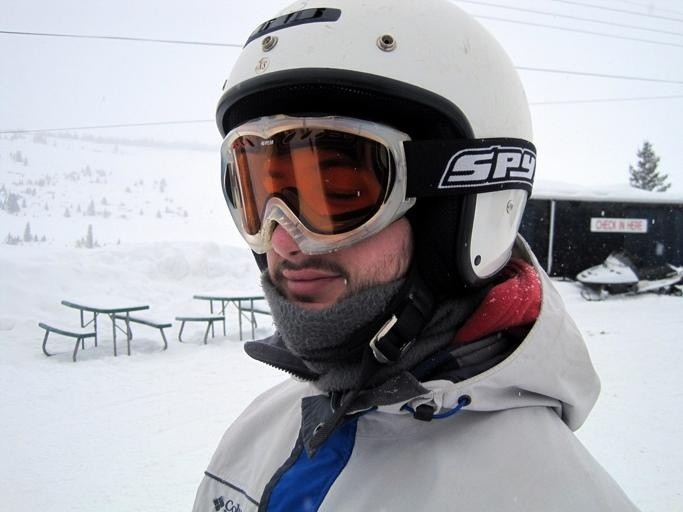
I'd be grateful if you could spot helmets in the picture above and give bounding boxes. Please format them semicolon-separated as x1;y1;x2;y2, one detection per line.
215;1;538;302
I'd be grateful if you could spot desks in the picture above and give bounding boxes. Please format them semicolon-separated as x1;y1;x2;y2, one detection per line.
61;300;149;356
194;296;265;341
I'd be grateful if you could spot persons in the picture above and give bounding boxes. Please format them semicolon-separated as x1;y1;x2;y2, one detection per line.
190;0;644;512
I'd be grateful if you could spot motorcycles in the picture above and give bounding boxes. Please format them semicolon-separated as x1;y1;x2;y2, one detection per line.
577;246;683;300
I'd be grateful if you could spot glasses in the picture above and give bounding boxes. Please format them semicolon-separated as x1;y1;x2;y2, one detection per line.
217;112;536;257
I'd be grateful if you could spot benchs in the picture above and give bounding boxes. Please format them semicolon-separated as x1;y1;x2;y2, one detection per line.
39;308;272;362
